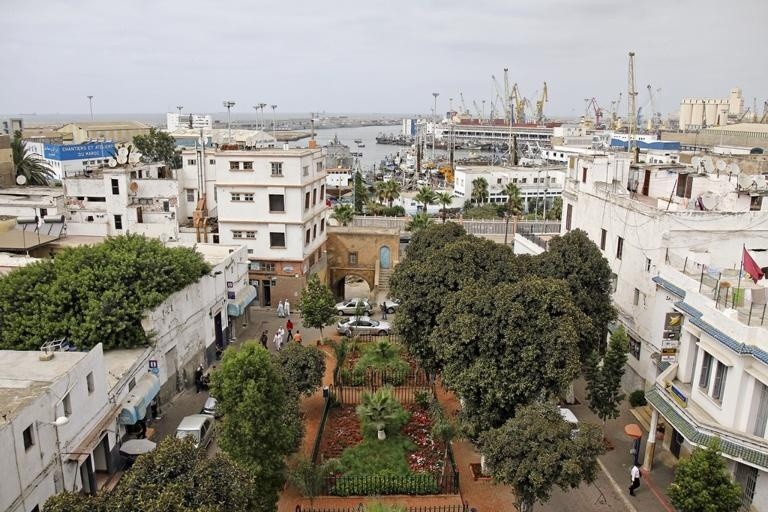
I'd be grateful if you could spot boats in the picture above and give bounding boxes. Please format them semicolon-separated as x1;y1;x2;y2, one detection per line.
455;142;544;167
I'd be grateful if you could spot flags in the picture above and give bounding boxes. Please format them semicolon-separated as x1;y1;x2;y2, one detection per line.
742;246;763;284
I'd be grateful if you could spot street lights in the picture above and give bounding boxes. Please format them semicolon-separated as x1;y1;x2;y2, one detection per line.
224;101;235;144
271;104;277;148
254;103;267;148
433;92;439;166
177;105;183;117
88;96;93;120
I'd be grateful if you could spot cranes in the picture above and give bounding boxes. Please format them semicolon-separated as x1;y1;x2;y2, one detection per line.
450;69;550;125
582;51;662;165
732;98;768;124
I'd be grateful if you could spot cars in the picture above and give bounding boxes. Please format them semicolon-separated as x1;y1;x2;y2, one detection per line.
203;396;220;416
175;414;215;449
336;316;391;338
335;298;375;316
380;299;402;314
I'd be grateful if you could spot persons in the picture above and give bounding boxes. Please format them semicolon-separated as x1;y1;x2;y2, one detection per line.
193;362;216;393
259;332;267;349
277;301;285;318
279;325;284;344
273;333;282;350
216;343;223;357
284;298;290;315
286;320;293;342
294;330;302;343
381;301;387;319
135;414;149;440
627;462;641;496
